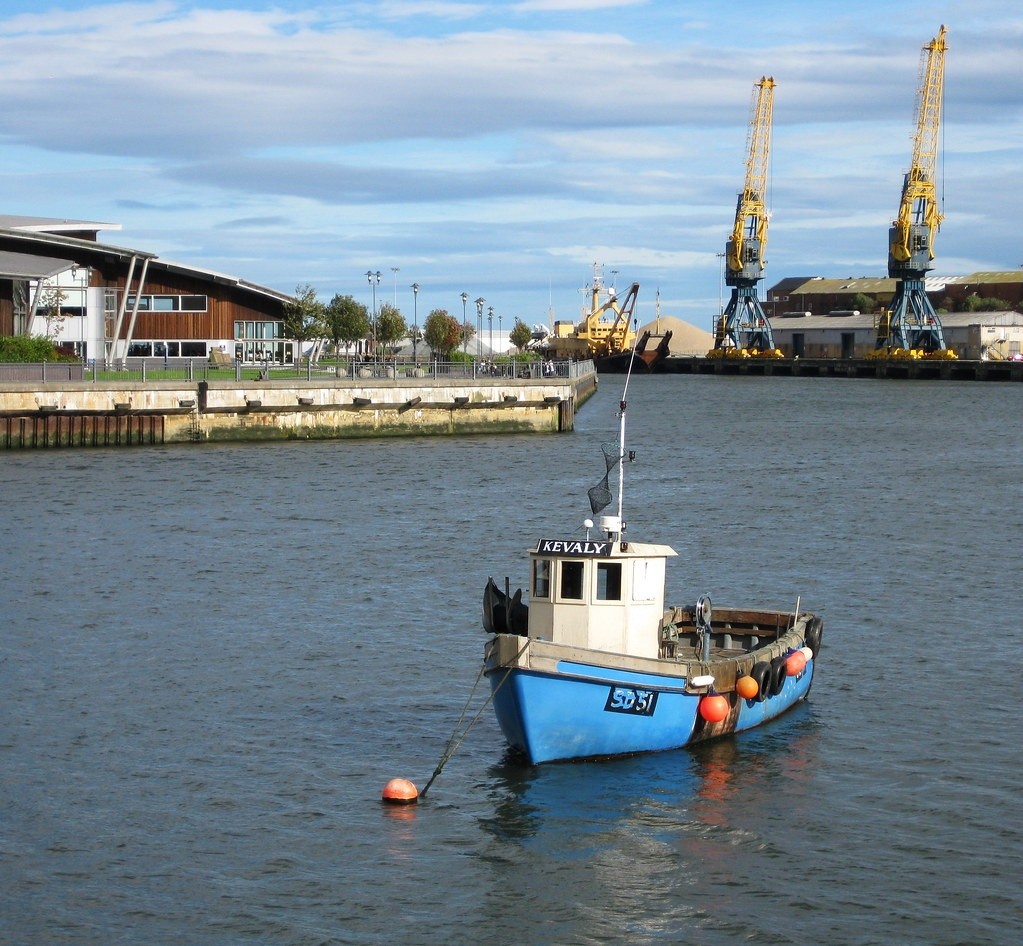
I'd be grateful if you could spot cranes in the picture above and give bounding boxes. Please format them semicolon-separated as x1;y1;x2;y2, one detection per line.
706;70;784;358
862;22;957;360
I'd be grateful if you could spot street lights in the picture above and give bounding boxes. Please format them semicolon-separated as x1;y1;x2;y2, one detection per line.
473;298;479;362
513;316;519;329
410;282;419;366
365;270;383;376
497;315;504;356
477;296;486;375
460;291;468;375
489;306;495;363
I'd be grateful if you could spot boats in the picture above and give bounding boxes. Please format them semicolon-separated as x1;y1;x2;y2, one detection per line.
479;335;827;773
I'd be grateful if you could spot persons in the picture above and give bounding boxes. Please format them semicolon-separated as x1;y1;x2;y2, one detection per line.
480;358;569;378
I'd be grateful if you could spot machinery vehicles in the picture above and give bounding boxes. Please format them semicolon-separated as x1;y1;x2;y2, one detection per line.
551;262;641;362
